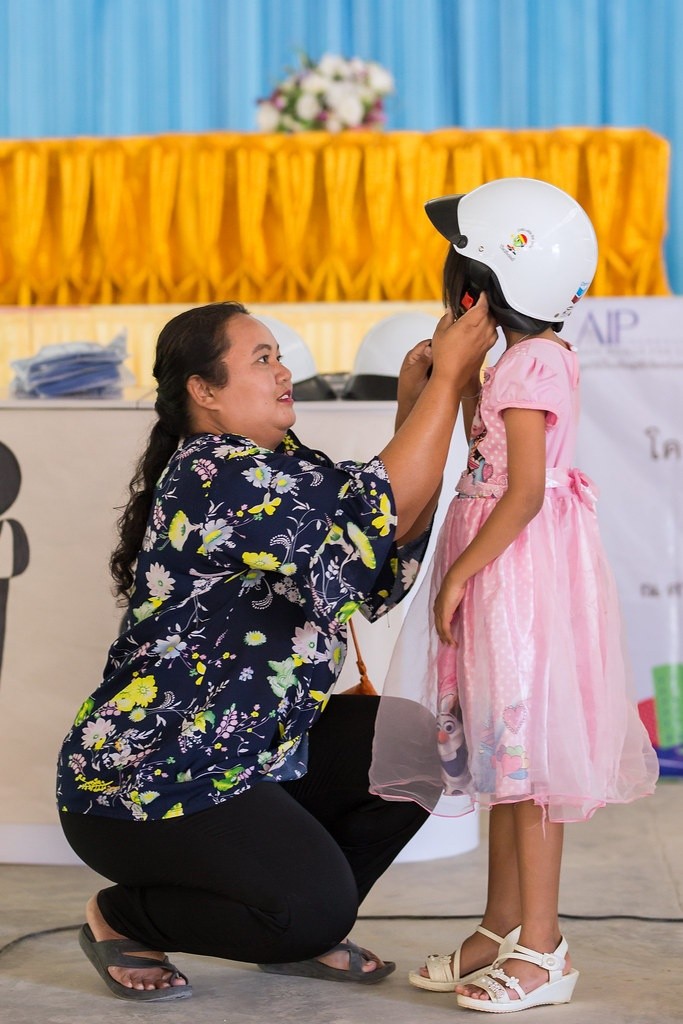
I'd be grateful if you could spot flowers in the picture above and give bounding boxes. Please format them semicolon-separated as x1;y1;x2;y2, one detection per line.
255;52;394;134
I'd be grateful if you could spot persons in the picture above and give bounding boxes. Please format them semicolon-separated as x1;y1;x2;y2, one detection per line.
370;173;659;1011
58;292;502;1003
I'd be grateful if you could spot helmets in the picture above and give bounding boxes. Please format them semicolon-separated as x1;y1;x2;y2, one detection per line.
424;177;598;323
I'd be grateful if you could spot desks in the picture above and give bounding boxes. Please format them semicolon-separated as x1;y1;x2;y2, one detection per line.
0;403;481;866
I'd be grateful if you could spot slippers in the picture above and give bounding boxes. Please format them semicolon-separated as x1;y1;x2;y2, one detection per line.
76;922;194;1003
256;938;397;984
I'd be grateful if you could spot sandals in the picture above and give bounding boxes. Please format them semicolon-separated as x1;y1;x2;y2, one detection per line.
453;932;580;1014
406;923;522;992
